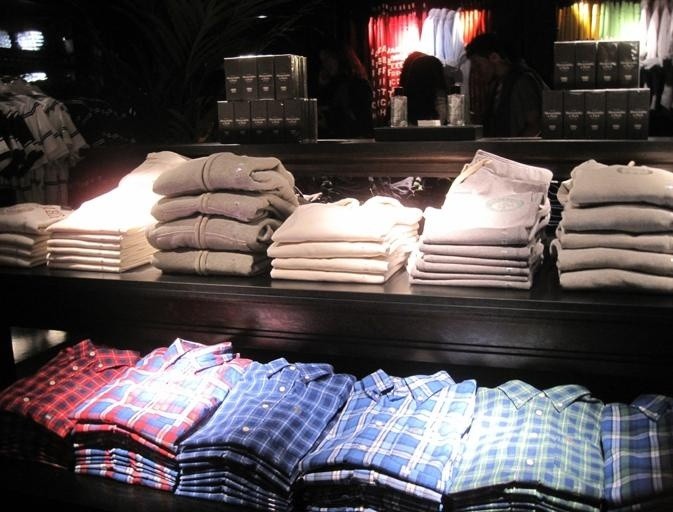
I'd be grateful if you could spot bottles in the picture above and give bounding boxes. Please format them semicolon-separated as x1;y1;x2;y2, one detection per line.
389;87;408;129
445;84;465;127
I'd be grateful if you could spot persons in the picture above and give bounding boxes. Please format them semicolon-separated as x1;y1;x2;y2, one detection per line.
313;38;377;140
386;53;444;125
459;31;550;138
